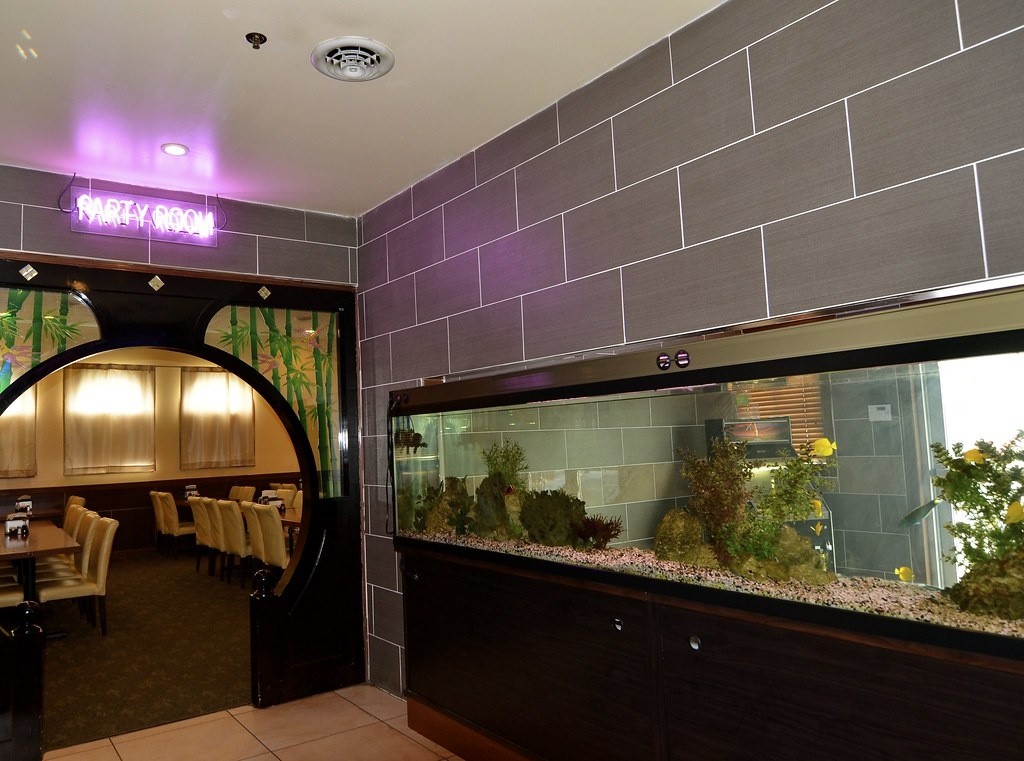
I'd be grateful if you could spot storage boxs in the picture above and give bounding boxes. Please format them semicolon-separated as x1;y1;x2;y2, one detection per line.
385;278;1024;666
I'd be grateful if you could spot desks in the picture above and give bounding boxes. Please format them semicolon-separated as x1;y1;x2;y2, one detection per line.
0;520;58;534
0;528;82;641
175;498;244;517
280;507;302;557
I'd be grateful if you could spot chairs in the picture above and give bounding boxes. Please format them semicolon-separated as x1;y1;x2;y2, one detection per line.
0;495;119;648
150;480;304;592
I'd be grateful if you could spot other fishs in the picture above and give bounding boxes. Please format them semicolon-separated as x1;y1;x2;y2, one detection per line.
812;438;838;457
895;567;915;581
964;449;988;464
1004;501;1024;524
810;500;822;536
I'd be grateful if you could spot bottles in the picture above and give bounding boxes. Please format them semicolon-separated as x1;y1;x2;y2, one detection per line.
280;499;286;517
21;518;29;540
